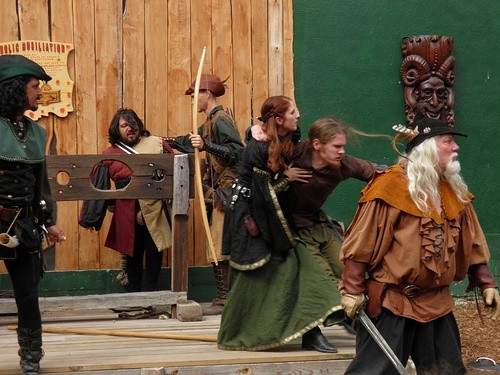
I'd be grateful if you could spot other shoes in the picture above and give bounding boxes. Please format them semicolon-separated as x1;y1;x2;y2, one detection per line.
112;305;157;319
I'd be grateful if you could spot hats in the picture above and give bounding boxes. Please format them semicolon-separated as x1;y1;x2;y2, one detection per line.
392;111;468;163
0;54;52;83
185;74;231;97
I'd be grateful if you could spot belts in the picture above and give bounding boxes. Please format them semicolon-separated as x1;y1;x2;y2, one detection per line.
385;283;450;298
0;203;37;221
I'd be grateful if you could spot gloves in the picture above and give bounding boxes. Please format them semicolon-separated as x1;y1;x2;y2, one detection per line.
483;287;500;320
340;293;366;318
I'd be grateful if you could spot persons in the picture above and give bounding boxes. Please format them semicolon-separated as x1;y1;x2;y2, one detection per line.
0;53;64;375
79;107;174;315
338;117;500;375
169;73;246;315
217;96;384;375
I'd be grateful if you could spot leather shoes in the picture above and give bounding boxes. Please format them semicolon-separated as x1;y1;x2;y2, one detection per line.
302;326;337;352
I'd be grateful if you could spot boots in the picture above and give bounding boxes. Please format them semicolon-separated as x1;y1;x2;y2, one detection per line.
15;328;43;375
203;261;229;316
